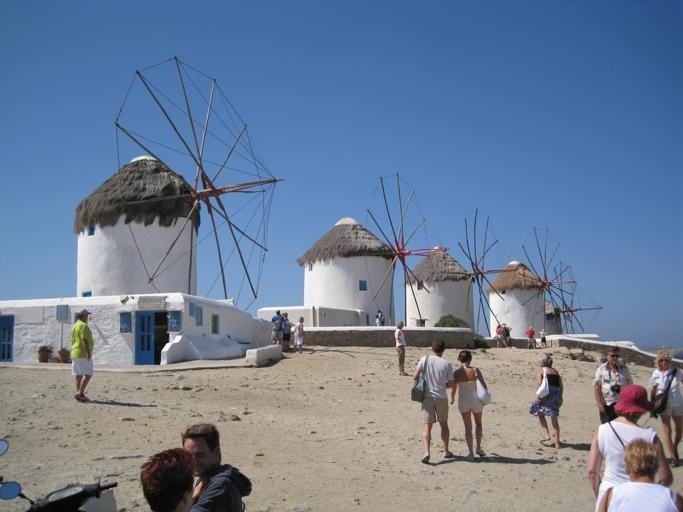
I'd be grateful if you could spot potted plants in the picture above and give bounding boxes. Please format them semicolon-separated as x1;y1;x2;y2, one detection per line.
58;349;71;362
38;345;53;362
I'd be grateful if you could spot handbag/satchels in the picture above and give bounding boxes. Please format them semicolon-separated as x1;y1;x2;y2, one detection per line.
650;392;667;413
475;378;491;405
535;366;550;399
411;376;426;402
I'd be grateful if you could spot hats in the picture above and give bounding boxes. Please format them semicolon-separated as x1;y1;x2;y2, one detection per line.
613;385;654;413
76;308;91;317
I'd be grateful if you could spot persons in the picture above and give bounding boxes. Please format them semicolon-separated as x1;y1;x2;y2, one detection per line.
646;347;682;468
592;344;634;425
271;309;305;353
375;314;382;327
393;320;410;377
587;386;676;499
377;310;385;327
69;308;95;402
182;422;252;511
141;448;194;512
595;440;682;511
450;349;490;460
495;322;548;349
536;355;563;450
412;338;456;464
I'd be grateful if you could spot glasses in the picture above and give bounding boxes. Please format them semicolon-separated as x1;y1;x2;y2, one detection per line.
611;356;619;358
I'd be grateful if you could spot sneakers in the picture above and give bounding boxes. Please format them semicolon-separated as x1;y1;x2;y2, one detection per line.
464;448;486;462
443;452;452;458
421;452;430;463
